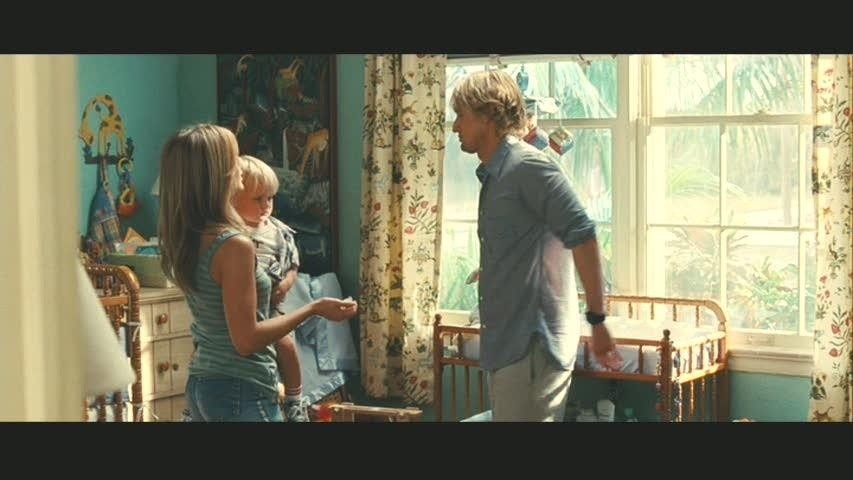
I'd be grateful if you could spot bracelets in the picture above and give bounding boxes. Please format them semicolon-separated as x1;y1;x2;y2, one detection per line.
585;311;604;325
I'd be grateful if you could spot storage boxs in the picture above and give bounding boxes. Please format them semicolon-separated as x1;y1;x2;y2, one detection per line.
108;252;176;288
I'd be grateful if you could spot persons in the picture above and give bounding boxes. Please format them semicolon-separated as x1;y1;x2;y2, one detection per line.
231;155;309;423
448;69;623;423
155;122;358;423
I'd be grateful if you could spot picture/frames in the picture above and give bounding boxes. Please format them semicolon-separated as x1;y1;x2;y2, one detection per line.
216;53;339;279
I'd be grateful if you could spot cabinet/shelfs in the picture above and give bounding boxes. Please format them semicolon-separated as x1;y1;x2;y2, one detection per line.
95;288;196;421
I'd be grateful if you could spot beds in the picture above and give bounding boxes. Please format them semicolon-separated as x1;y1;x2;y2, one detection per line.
80;263;143;422
433;292;729;422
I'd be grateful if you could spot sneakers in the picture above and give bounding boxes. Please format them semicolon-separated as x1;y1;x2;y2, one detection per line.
284;395;312;423
